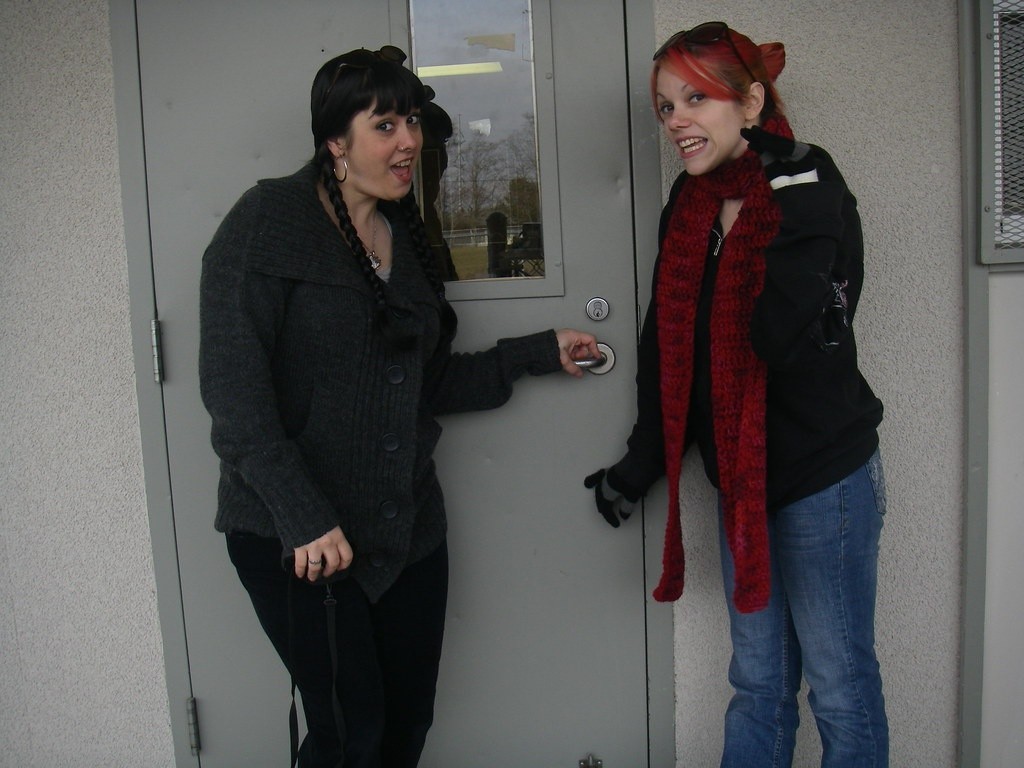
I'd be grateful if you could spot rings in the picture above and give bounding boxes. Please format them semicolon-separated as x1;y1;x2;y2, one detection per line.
308;559;321;564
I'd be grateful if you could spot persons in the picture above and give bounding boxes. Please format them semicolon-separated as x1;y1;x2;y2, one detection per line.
585;21;888;767
201;46;599;767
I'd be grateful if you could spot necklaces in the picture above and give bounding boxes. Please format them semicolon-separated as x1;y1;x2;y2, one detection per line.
354;214;382;271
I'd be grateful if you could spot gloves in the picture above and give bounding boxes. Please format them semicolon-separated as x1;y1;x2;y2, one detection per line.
739;125;820;195
583;456;656;528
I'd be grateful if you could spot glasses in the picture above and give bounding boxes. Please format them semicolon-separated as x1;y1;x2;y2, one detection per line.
321;45;407;107
423;85;435;100
653;21;758;83
